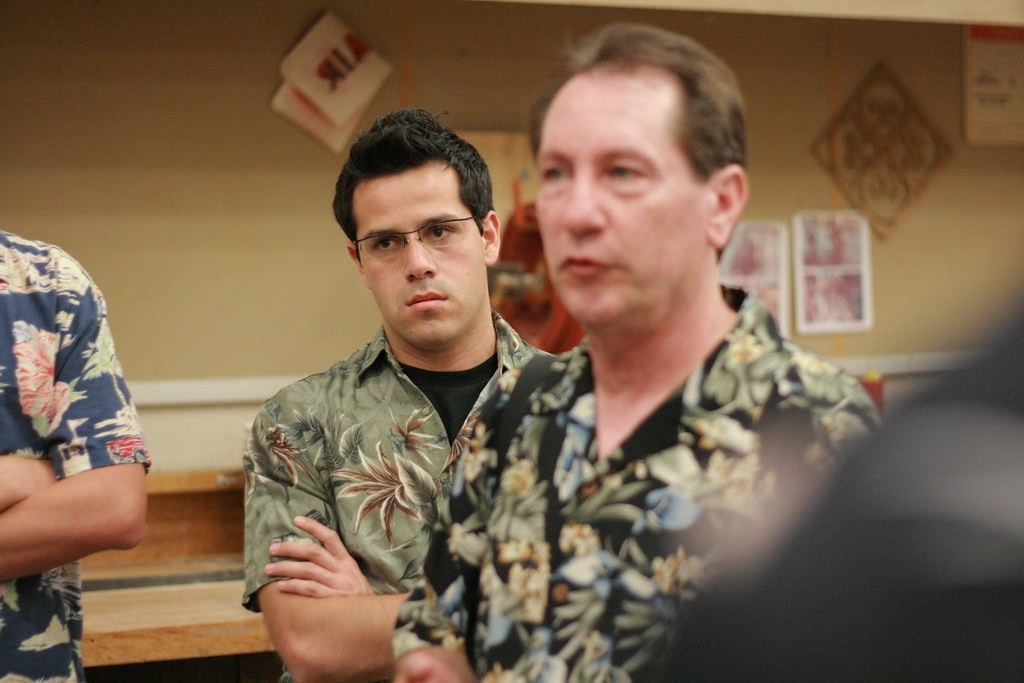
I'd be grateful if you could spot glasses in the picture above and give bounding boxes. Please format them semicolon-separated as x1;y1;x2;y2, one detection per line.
354;216;477;260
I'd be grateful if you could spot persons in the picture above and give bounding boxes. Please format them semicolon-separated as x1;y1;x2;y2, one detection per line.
0;231;153;683
240;105;553;683
389;22;887;683
